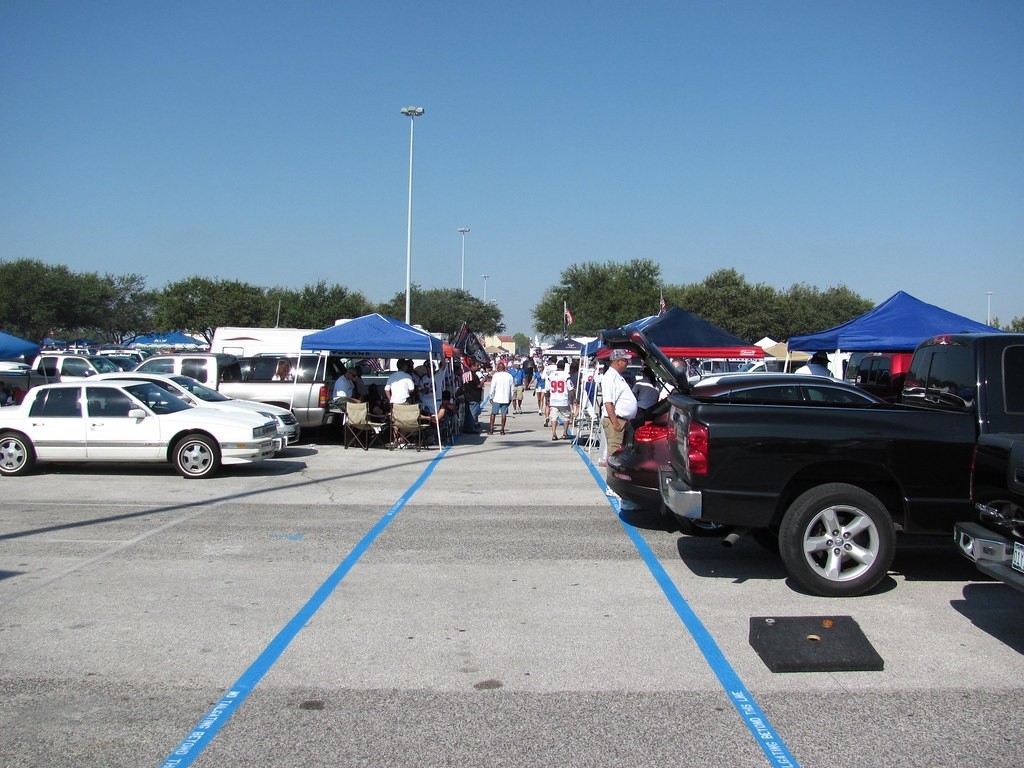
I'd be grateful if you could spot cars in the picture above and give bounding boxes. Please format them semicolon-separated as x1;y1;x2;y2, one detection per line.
588;364;654;395
0;345;356;480
0;380;279;479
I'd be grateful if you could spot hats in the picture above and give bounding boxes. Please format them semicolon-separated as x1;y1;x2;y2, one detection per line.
609;349;632;360
815;352;831;362
514;361;523;365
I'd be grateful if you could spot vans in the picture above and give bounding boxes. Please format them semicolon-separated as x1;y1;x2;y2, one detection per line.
696;356;810;381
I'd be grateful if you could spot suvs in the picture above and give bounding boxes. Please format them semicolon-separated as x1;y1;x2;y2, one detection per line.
593;324;900;537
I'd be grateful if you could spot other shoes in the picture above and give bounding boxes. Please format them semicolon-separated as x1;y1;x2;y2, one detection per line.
544;417;549;427
391;442;407;449
500;429;505;435
606;486;619;497
488;429;492;434
518;407;522;413
513;410;516;413
462;430;479;434
539;410;542;416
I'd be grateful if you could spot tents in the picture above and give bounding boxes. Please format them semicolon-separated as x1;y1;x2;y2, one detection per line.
442;342;463;406
0;332;210;383
290;312;454;454
545;290;1010;455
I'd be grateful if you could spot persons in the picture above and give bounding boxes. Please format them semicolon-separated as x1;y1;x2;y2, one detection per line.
333;358;485;448
794;352;836;402
0;381;27;406
487;352;581;441
598;346;720;468
272;360;293;381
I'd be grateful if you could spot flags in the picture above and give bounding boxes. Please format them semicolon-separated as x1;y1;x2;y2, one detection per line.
451;323;491;368
356;359;384;372
565;309;572;332
661;298;666;313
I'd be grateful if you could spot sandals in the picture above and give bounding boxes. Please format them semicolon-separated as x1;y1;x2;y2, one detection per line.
552;436;559;440
562;435;574;439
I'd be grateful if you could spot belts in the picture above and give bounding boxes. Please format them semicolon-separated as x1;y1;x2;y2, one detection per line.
605;415;627;420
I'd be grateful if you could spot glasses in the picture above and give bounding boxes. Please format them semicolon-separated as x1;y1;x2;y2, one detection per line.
623;359;629;363
350;371;356;377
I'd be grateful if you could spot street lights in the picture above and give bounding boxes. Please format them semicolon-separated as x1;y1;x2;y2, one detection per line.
400;105;424;326
456;227;471;292
482;275;490;305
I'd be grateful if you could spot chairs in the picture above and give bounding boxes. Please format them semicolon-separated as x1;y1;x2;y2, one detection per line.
323;381;457;452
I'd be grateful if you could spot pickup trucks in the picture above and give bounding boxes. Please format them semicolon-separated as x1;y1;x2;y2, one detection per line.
654;329;1024;598
